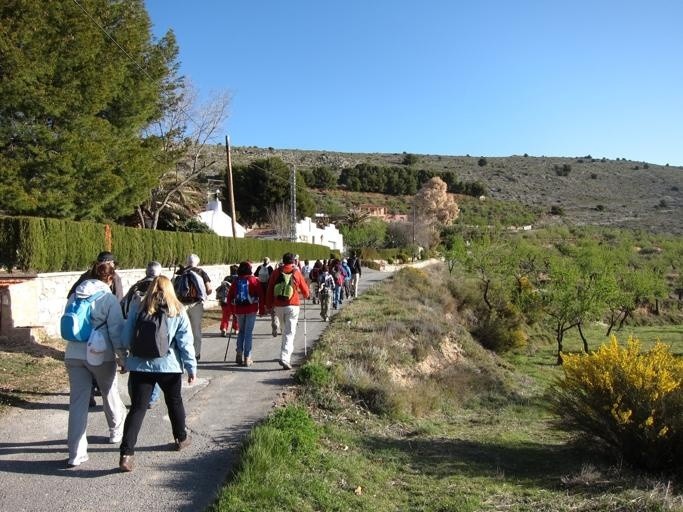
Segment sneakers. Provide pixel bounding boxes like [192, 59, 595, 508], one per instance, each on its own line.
[175, 435, 193, 452]
[235, 354, 242, 363]
[244, 356, 254, 367]
[69, 455, 89, 466]
[119, 454, 135, 473]
[221, 328, 225, 336]
[279, 359, 292, 368]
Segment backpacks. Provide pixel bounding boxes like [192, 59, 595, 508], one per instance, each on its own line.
[230, 277, 259, 306]
[258, 264, 270, 283]
[61, 291, 104, 342]
[215, 281, 230, 304]
[132, 301, 171, 358]
[273, 269, 301, 301]
[172, 266, 201, 303]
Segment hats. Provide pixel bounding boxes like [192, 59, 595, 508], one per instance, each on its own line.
[98, 251, 119, 262]
[86, 328, 106, 366]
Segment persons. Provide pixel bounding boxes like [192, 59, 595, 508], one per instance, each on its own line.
[61, 250, 363, 473]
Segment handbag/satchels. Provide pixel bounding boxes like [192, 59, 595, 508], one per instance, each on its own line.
[320, 287, 333, 297]
